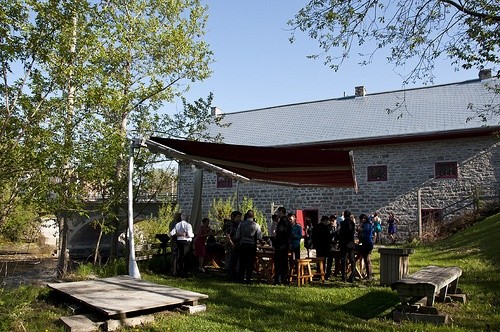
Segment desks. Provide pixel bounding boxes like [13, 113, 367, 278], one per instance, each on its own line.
[332, 250, 362, 276]
[204, 242, 225, 270]
[378, 248, 414, 286]
[257, 252, 292, 283]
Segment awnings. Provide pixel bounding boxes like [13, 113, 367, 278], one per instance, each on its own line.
[128, 136, 358, 279]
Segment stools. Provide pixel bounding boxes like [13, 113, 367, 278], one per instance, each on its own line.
[290, 258, 325, 285]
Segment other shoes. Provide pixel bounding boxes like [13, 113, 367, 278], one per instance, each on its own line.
[341, 277, 346, 282]
[350, 276, 356, 283]
[198, 266, 206, 272]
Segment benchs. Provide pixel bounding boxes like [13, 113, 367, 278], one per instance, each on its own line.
[390, 265, 466, 324]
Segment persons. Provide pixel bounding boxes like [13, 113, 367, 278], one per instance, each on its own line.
[169, 207, 398, 286]
